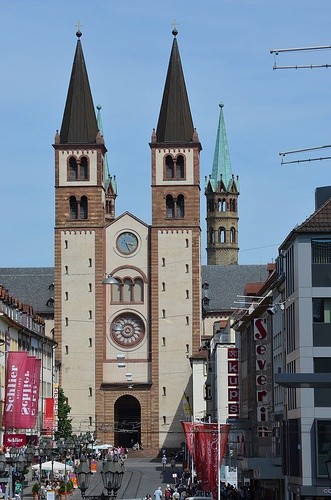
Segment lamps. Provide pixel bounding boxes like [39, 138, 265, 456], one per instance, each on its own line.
[102, 275, 118, 285]
[48, 283, 55, 291]
[202, 281, 210, 290]
[202, 295, 210, 306]
[45, 297, 54, 307]
[267, 306, 278, 315]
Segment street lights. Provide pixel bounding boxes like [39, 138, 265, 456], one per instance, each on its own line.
[10, 432, 100, 500]
[74, 454, 126, 500]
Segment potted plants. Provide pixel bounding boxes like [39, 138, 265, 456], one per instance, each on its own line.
[59, 480, 75, 500]
[31, 483, 40, 500]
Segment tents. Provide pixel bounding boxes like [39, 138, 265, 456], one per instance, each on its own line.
[32, 460, 73, 480]
[85, 443, 112, 453]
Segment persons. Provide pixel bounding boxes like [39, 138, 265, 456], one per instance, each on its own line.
[0, 446, 129, 500]
[133, 442, 260, 500]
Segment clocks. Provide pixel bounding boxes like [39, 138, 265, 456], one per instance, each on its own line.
[112, 228, 141, 257]
